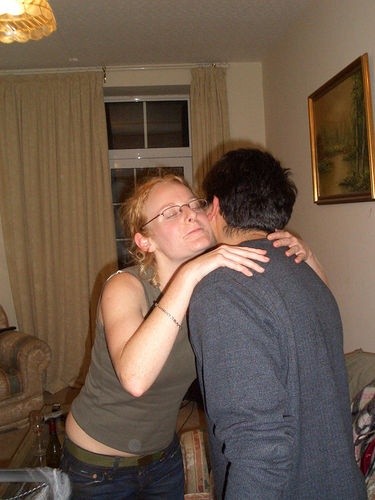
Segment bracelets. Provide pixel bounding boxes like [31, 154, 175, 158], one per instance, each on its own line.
[155, 303, 182, 329]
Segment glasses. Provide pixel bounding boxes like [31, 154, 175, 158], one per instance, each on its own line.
[143, 198, 208, 228]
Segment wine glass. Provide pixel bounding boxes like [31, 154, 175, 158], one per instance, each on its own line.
[30, 410, 46, 456]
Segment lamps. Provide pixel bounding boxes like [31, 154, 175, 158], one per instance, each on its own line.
[0, 0, 59, 46]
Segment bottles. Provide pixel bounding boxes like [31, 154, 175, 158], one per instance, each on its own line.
[48, 418, 61, 455]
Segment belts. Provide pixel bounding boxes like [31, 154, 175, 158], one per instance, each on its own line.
[64, 432, 164, 466]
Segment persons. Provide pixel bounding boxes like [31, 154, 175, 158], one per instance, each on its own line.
[60, 173, 328, 500]
[189, 147, 368, 500]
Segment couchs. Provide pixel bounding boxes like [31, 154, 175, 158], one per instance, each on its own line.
[178, 349, 375, 500]
[0, 305, 52, 433]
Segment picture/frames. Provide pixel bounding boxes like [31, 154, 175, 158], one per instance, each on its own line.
[308, 53, 375, 205]
